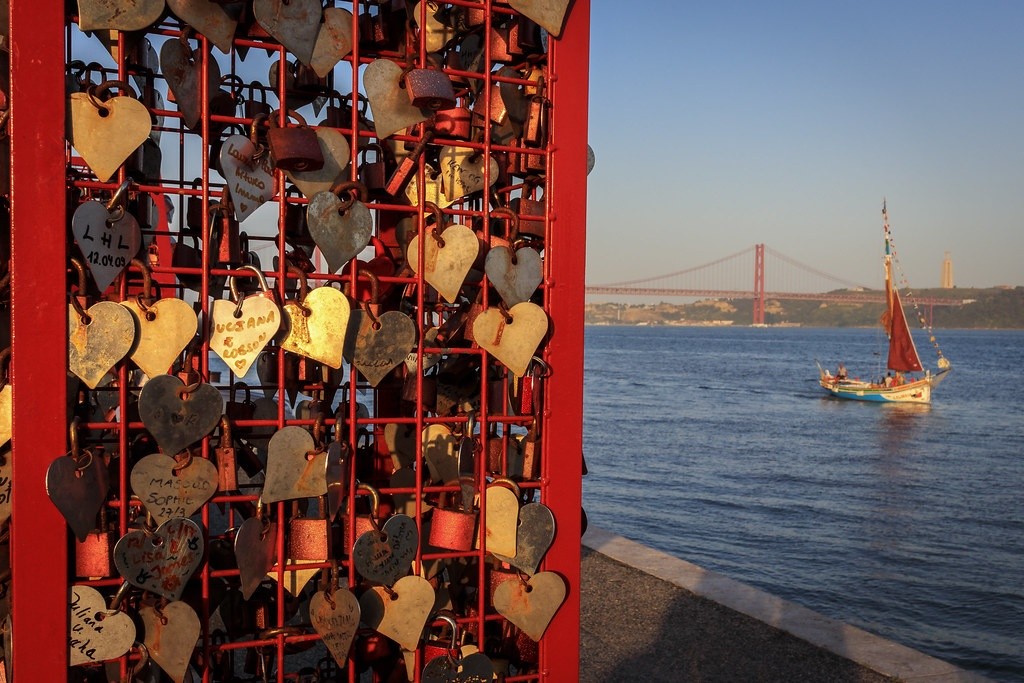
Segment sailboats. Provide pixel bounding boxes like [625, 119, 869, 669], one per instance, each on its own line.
[815, 193, 953, 403]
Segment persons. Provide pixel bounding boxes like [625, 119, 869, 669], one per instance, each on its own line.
[885, 371, 903, 388]
[835, 364, 848, 384]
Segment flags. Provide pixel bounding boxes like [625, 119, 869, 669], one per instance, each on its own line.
[888, 230, 943, 360]
